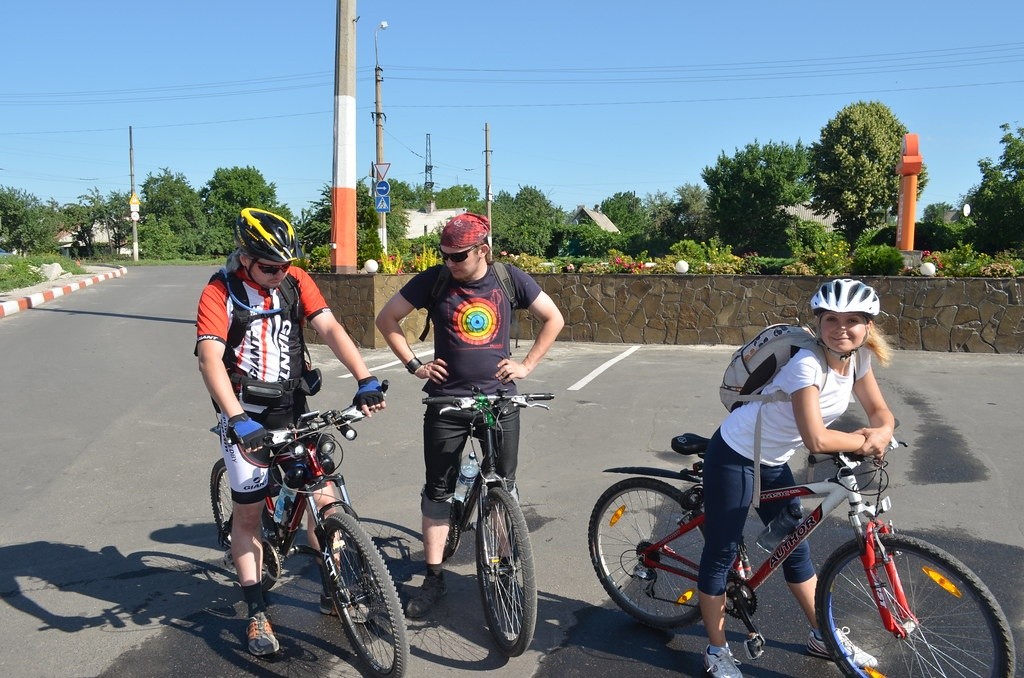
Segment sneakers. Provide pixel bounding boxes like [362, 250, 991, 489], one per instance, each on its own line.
[497, 570, 526, 609]
[806, 626, 878, 668]
[703, 642, 742, 678]
[320, 596, 369, 623]
[405, 573, 448, 618]
[245, 612, 280, 655]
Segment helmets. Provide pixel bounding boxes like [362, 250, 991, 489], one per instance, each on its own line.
[810, 279, 880, 316]
[235, 208, 303, 263]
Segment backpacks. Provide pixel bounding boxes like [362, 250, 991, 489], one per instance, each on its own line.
[718, 323, 857, 508]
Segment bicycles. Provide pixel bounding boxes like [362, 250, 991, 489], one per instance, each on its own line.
[419, 383, 555, 658]
[585, 432, 1017, 678]
[209, 377, 411, 678]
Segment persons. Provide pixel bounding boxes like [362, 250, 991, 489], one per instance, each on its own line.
[193, 207, 387, 657]
[375, 213, 564, 618]
[697, 277, 895, 678]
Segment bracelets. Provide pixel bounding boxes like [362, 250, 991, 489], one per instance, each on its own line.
[405, 357, 424, 374]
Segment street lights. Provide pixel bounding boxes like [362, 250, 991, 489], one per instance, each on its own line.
[371, 20, 390, 256]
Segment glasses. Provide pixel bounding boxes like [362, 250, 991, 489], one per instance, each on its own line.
[439, 247, 477, 262]
[257, 263, 291, 273]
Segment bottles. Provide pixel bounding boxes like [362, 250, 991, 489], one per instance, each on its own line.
[755, 503, 804, 553]
[272, 467, 302, 525]
[453, 452, 480, 502]
